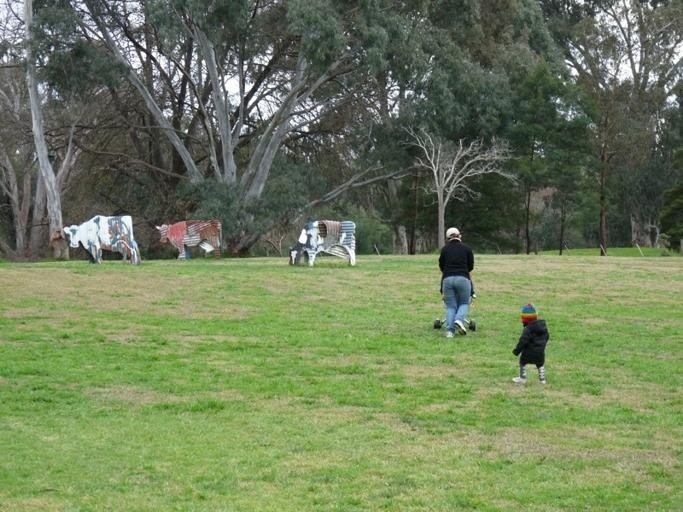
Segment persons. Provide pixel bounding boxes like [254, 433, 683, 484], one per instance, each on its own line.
[512, 303, 550, 387]
[439, 227, 475, 339]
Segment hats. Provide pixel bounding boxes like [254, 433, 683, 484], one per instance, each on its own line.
[446, 227, 460, 239]
[521, 302, 538, 322]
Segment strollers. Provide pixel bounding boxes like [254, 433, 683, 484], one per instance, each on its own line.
[430, 270, 480, 335]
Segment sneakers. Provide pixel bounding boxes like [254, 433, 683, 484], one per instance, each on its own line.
[512, 376, 527, 385]
[536, 378, 546, 385]
[445, 330, 455, 338]
[454, 320, 467, 335]
